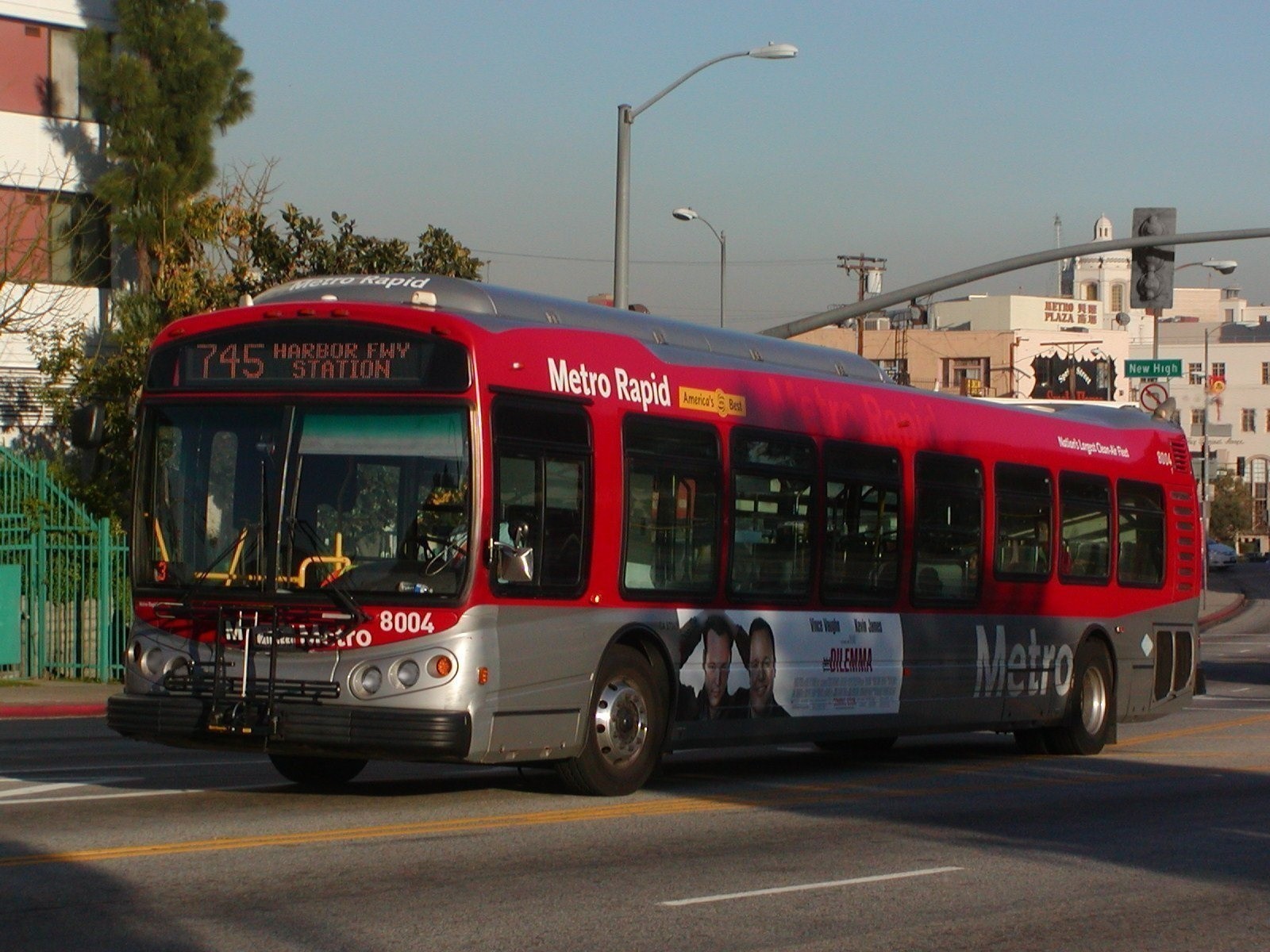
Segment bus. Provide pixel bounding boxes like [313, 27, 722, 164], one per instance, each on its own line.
[68, 277, 1207, 797]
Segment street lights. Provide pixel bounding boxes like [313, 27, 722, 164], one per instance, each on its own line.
[673, 209, 726, 331]
[611, 42, 800, 314]
[1149, 258, 1237, 377]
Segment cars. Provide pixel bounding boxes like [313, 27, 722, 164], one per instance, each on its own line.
[1207, 536, 1237, 570]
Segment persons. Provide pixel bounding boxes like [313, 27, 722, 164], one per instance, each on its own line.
[443, 488, 516, 583]
[677, 609, 753, 722]
[1033, 516, 1072, 575]
[965, 550, 979, 582]
[739, 616, 792, 719]
